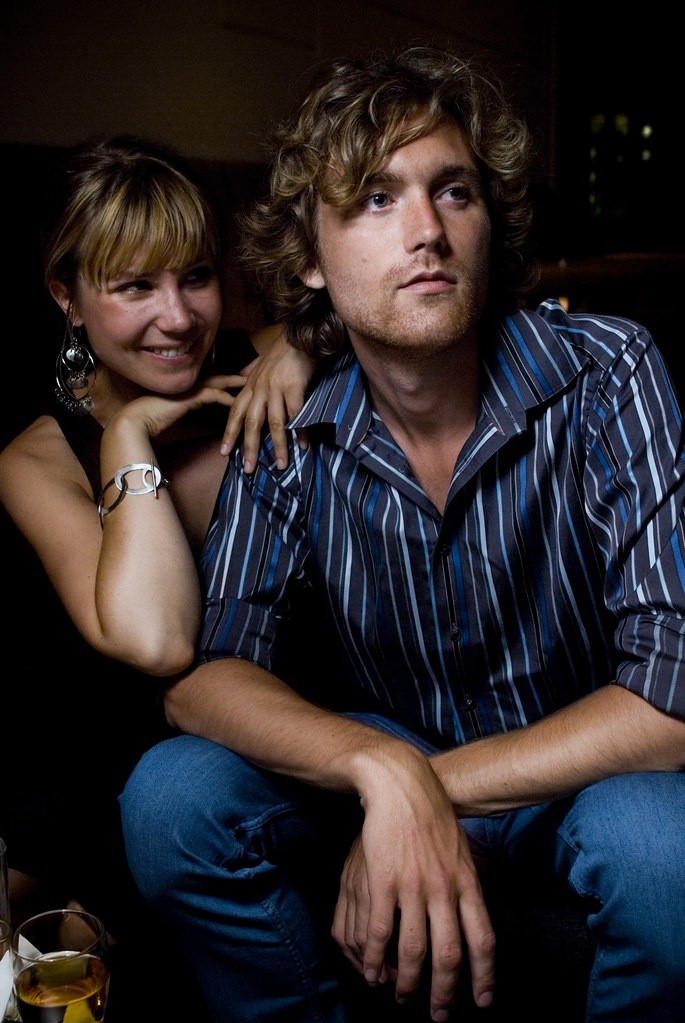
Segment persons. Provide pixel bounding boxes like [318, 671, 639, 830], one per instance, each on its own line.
[115, 41, 685, 1023]
[0, 135, 318, 1023]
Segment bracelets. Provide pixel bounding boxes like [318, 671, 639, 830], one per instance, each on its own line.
[97, 458, 171, 528]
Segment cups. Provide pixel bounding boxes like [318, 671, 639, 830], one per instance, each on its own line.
[9, 909, 111, 1023]
[0, 918, 22, 1023]
